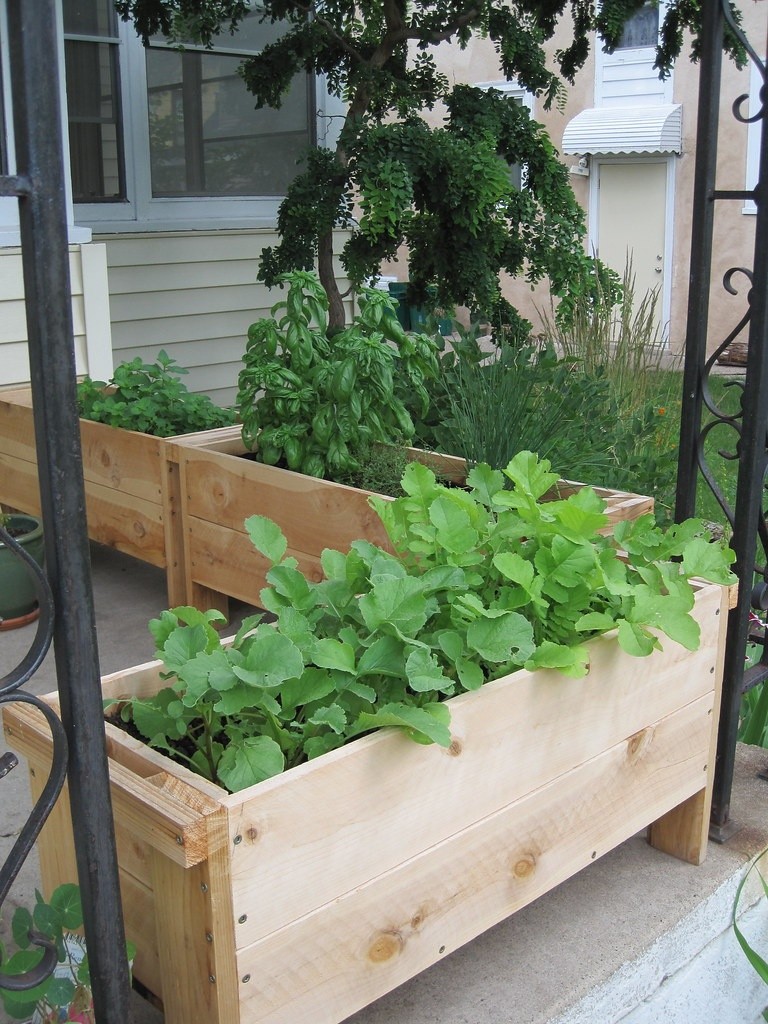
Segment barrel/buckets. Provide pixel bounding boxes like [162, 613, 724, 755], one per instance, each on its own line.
[380, 282, 411, 330]
[410, 286, 452, 336]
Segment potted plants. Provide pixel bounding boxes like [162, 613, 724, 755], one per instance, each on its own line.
[165, 268, 656, 628]
[2, 451, 739, 1024]
[1, 349, 244, 618]
